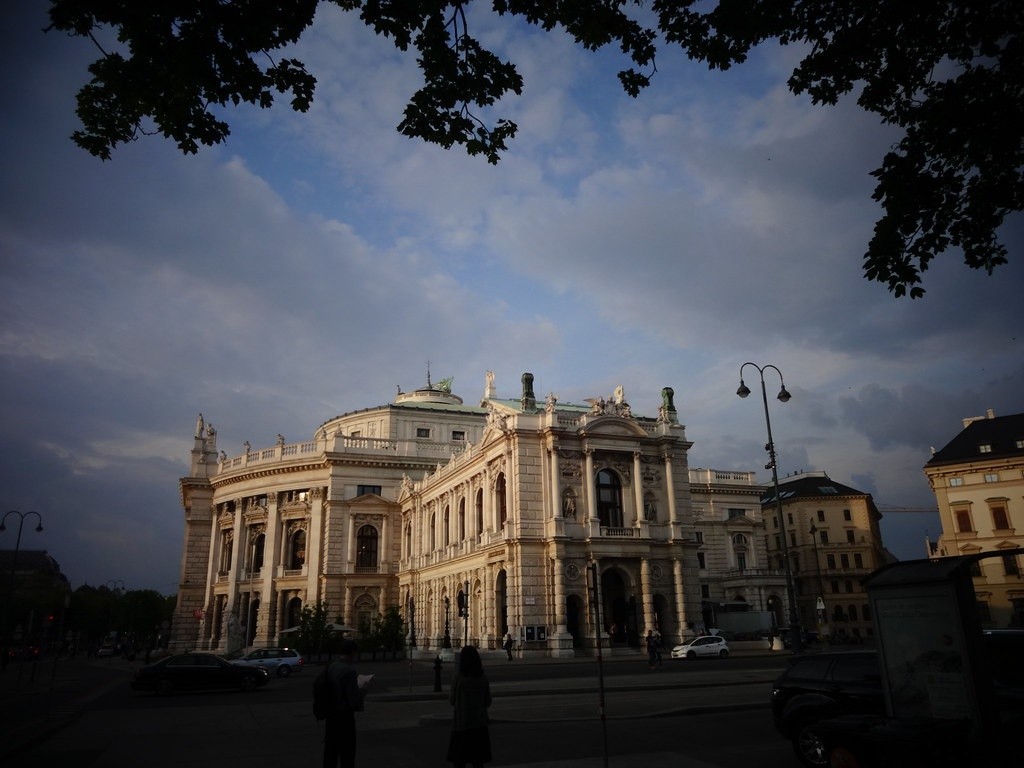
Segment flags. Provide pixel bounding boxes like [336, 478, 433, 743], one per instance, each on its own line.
[193, 610, 201, 619]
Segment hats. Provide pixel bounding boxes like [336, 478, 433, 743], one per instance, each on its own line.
[655, 634, 660, 637]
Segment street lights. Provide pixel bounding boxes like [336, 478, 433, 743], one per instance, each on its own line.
[441, 596, 453, 649]
[736, 361, 810, 657]
[102, 578, 128, 637]
[1, 510, 44, 622]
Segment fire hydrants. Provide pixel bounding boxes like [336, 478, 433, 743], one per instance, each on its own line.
[434, 655, 445, 693]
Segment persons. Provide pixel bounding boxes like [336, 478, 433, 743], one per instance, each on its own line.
[699, 628, 712, 636]
[505, 634, 513, 661]
[313, 645, 375, 768]
[607, 622, 664, 669]
[447, 645, 493, 768]
[195, 369, 659, 524]
[88, 639, 136, 664]
[768, 632, 774, 650]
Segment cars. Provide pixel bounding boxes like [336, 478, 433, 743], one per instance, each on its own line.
[671, 635, 731, 659]
[130, 652, 272, 698]
[226, 646, 304, 678]
[766, 627, 1024, 767]
[98, 644, 114, 657]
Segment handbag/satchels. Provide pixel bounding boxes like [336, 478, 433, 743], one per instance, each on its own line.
[312, 673, 332, 721]
